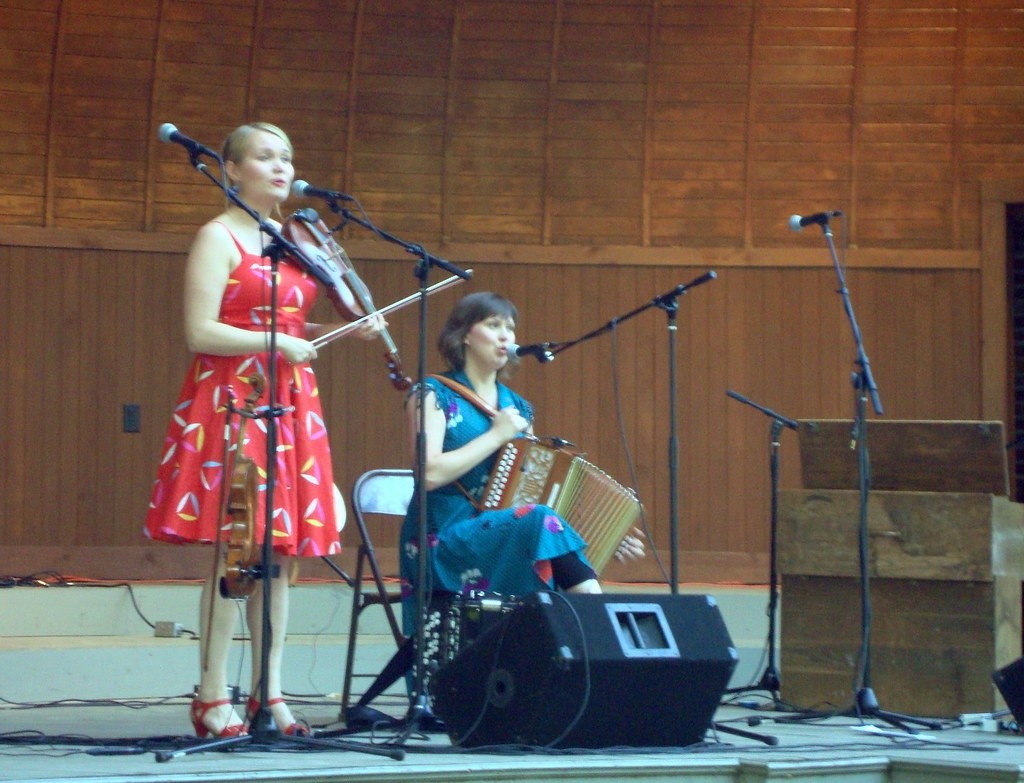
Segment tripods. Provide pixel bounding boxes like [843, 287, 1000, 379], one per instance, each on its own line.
[722, 223, 944, 735]
[153, 155, 473, 763]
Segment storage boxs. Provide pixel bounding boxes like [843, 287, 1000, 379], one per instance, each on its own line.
[774, 422, 1023, 723]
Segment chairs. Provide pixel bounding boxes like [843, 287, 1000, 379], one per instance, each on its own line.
[340, 467, 418, 733]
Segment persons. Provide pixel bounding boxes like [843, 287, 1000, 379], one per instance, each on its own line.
[141, 120, 391, 743]
[397, 291, 647, 719]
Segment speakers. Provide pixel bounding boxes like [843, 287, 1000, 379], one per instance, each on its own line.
[428, 591, 738, 755]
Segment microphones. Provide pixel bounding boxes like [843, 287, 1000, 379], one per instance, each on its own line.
[505, 341, 553, 362]
[788, 210, 841, 232]
[291, 179, 354, 201]
[159, 122, 221, 162]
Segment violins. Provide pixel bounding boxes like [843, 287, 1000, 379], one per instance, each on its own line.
[279, 207, 415, 391]
[215, 370, 264, 600]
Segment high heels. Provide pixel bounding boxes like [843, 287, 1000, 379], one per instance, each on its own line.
[190, 699, 248, 737]
[246, 697, 309, 737]
